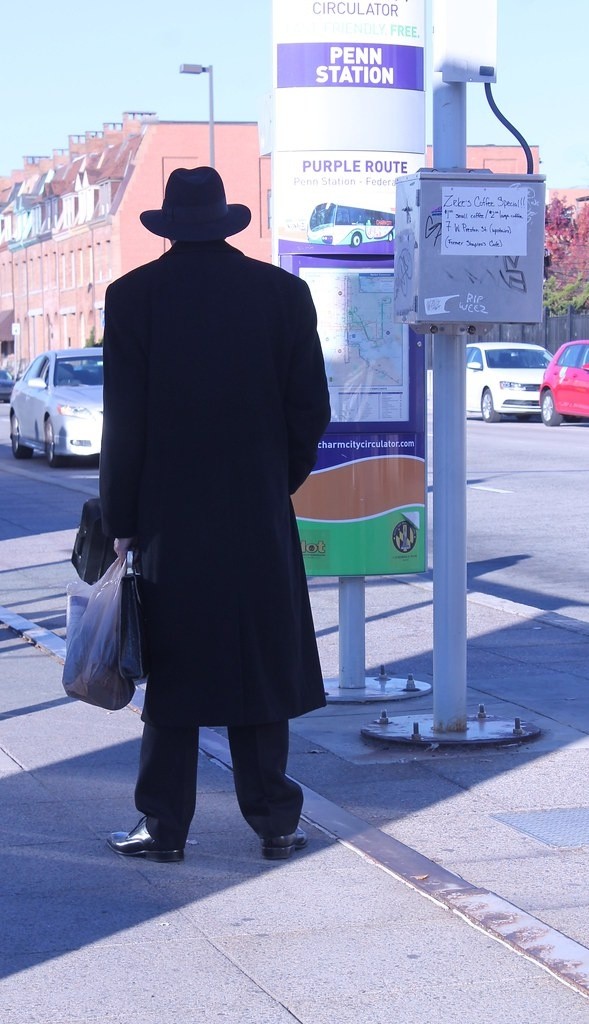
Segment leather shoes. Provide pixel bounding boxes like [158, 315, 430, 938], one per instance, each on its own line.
[261, 821, 309, 859]
[108, 817, 188, 862]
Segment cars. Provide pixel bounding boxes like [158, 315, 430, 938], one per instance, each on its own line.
[538, 339, 589, 427]
[0, 369, 15, 404]
[8, 347, 103, 468]
[465, 342, 555, 423]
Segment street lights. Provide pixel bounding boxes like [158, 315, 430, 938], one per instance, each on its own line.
[181, 63, 217, 169]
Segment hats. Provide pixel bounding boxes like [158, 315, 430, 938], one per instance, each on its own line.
[140, 167, 251, 239]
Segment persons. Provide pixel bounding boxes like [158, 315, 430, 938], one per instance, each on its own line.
[99, 166, 331, 863]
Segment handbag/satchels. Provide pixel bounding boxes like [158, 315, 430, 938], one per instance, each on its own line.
[72, 498, 133, 584]
[62, 550, 139, 709]
[116, 549, 147, 683]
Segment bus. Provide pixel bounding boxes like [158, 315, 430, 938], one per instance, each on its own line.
[306, 203, 396, 249]
